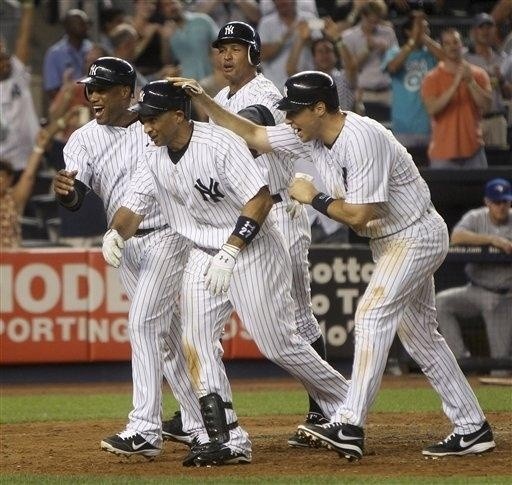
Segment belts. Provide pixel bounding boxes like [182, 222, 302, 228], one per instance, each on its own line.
[471, 280, 510, 295]
[482, 111, 502, 119]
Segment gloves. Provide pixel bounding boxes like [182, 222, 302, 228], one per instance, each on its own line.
[101, 228, 125, 269]
[285, 172, 313, 220]
[203, 243, 240, 296]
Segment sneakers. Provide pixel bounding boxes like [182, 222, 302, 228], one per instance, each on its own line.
[297, 421, 364, 462]
[287, 408, 324, 448]
[101, 429, 162, 458]
[161, 411, 193, 443]
[194, 442, 252, 466]
[421, 421, 496, 456]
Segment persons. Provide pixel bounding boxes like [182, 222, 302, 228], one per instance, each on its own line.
[100, 79, 351, 466]
[51, 56, 208, 458]
[285, 15, 359, 112]
[492, 1, 512, 21]
[0, 127, 51, 249]
[154, 19, 328, 448]
[167, 70, 498, 461]
[420, 25, 494, 169]
[124, 0, 163, 77]
[96, 7, 126, 57]
[57, 1, 113, 44]
[298, 0, 360, 31]
[160, 0, 220, 81]
[42, 8, 97, 101]
[462, 13, 512, 149]
[379, 10, 445, 146]
[340, 1, 399, 105]
[256, 0, 323, 98]
[44, 47, 110, 156]
[194, 0, 261, 32]
[108, 22, 182, 92]
[0, 0, 43, 169]
[436, 179, 512, 377]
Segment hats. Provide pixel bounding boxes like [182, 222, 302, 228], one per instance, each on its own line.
[485, 178, 512, 203]
[475, 13, 494, 28]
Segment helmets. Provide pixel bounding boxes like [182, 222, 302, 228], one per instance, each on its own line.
[76, 57, 135, 87]
[273, 71, 340, 112]
[212, 23, 261, 50]
[124, 80, 191, 117]
